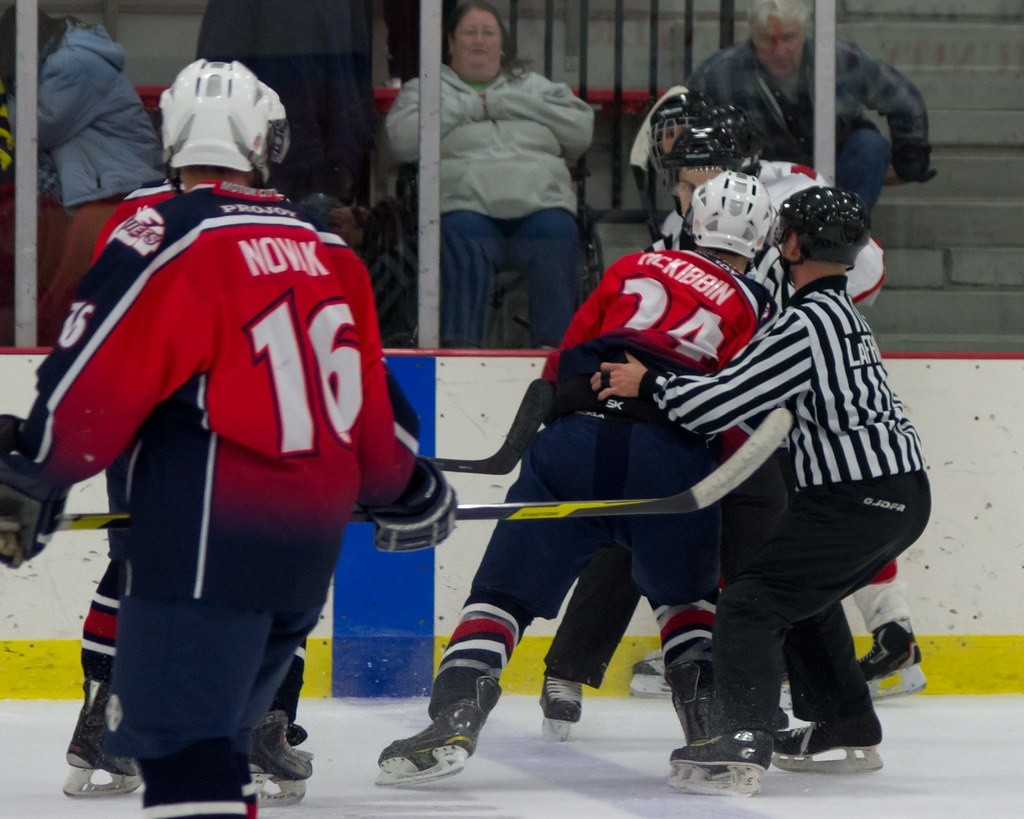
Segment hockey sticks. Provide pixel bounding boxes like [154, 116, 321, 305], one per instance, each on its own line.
[419, 377, 557, 476]
[2, 406, 792, 533]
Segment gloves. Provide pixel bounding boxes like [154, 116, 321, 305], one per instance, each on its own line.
[0, 452, 71, 571]
[371, 452, 461, 553]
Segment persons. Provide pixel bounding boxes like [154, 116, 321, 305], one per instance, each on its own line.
[687, 0, 940, 214]
[375, 98, 930, 794]
[0, 60, 457, 819]
[382, 0, 595, 355]
[1, 4, 166, 207]
[196, 0, 377, 211]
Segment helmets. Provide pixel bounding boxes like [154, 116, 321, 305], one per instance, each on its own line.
[650, 92, 762, 177]
[157, 58, 290, 192]
[772, 185, 872, 267]
[687, 170, 778, 260]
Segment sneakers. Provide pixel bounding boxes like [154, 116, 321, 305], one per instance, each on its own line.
[244, 710, 315, 808]
[64, 677, 141, 798]
[372, 674, 503, 790]
[767, 720, 885, 774]
[667, 716, 780, 797]
[538, 675, 584, 744]
[853, 619, 926, 700]
[630, 649, 676, 699]
[778, 675, 794, 712]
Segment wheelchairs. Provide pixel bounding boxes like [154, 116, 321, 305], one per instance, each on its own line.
[353, 102, 610, 342]
